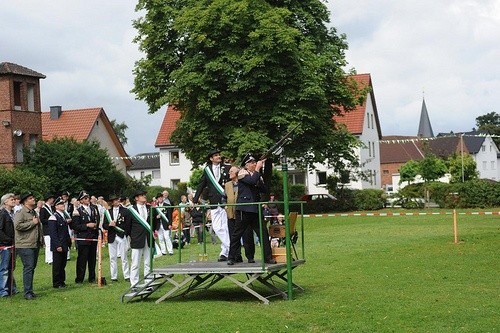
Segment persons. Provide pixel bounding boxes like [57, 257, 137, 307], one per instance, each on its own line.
[13, 192, 45, 300]
[273, 214, 294, 247]
[191, 149, 278, 264]
[0, 193, 20, 298]
[11, 195, 24, 214]
[34, 191, 108, 289]
[171, 193, 217, 249]
[102, 189, 174, 289]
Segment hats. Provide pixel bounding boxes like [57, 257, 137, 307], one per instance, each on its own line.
[54, 196, 66, 205]
[208, 149, 221, 158]
[18, 192, 33, 203]
[45, 194, 53, 201]
[155, 193, 163, 199]
[77, 191, 89, 201]
[133, 189, 148, 198]
[241, 153, 255, 166]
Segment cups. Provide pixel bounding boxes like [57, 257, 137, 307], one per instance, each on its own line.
[204, 255, 208, 261]
[199, 255, 202, 261]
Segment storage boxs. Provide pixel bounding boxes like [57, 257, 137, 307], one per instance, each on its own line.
[271, 246, 292, 263]
[269, 225, 286, 237]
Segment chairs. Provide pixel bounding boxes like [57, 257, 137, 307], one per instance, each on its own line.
[269, 212, 299, 260]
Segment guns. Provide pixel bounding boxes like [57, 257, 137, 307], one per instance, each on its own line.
[255, 122, 303, 163]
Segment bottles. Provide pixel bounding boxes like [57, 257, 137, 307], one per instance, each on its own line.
[104, 230, 108, 244]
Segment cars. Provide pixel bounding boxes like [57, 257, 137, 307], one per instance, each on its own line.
[384, 191, 426, 209]
[301, 193, 337, 201]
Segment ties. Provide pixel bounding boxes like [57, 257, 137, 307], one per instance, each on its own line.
[114, 209, 118, 222]
[141, 205, 146, 219]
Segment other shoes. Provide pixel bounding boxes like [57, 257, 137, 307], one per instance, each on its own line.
[218, 255, 227, 261]
[26, 291, 34, 300]
[264, 259, 277, 263]
[235, 257, 243, 262]
[146, 286, 153, 291]
[53, 283, 67, 288]
[227, 259, 234, 265]
[132, 287, 137, 292]
[248, 258, 255, 263]
